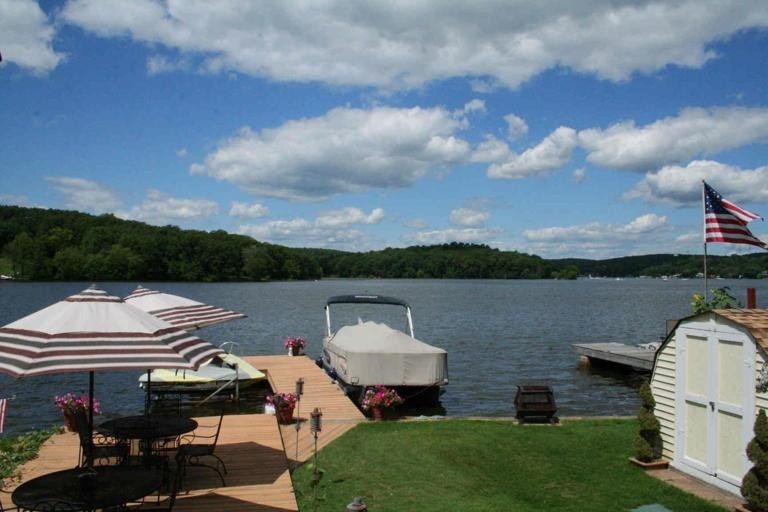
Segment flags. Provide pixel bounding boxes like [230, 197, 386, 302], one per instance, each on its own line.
[703, 180, 768, 250]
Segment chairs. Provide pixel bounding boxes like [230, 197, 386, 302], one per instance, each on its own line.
[0, 410, 228, 511]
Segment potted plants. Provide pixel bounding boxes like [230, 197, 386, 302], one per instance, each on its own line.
[734, 408, 768, 512]
[629, 381, 668, 470]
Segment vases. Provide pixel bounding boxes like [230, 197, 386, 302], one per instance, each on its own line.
[276, 408, 293, 424]
[290, 347, 298, 356]
[370, 406, 387, 420]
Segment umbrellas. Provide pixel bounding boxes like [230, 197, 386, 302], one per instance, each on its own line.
[120, 283, 248, 415]
[0, 280, 230, 468]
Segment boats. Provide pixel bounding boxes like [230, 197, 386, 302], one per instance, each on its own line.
[322, 294, 448, 392]
[137, 353, 267, 393]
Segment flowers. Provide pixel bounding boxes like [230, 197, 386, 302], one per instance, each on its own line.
[266, 392, 297, 417]
[361, 385, 405, 414]
[52, 389, 101, 417]
[283, 337, 305, 352]
